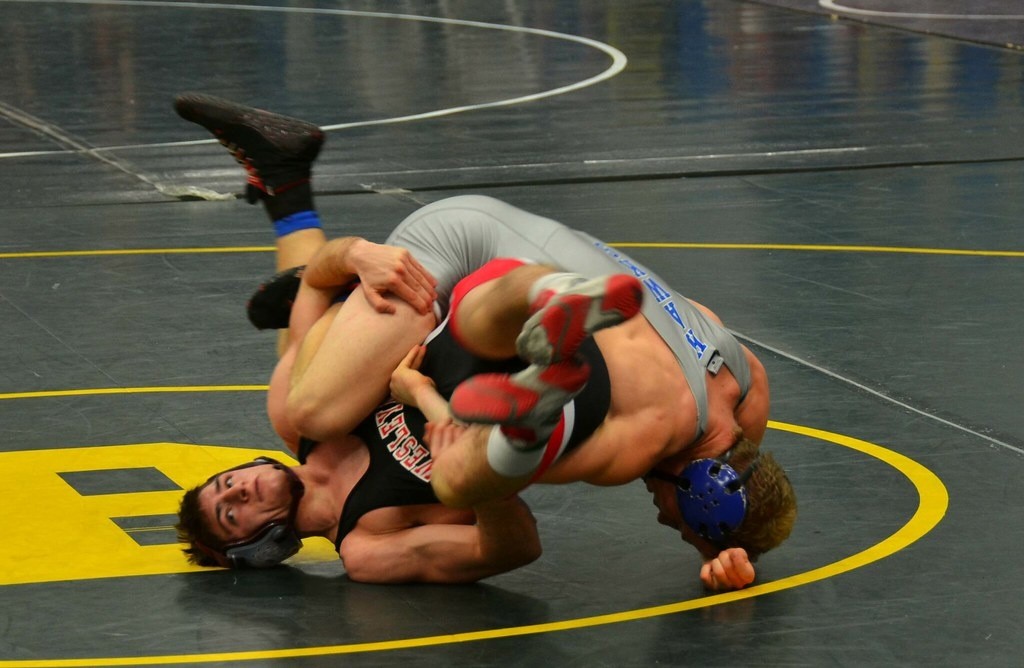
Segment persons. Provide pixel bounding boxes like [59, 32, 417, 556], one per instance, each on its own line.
[174, 236, 644, 584]
[171, 92, 797, 590]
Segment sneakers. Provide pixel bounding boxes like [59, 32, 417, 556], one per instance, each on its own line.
[449, 355, 592, 444]
[247, 264, 361, 330]
[515, 274, 644, 364]
[171, 90, 326, 205]
